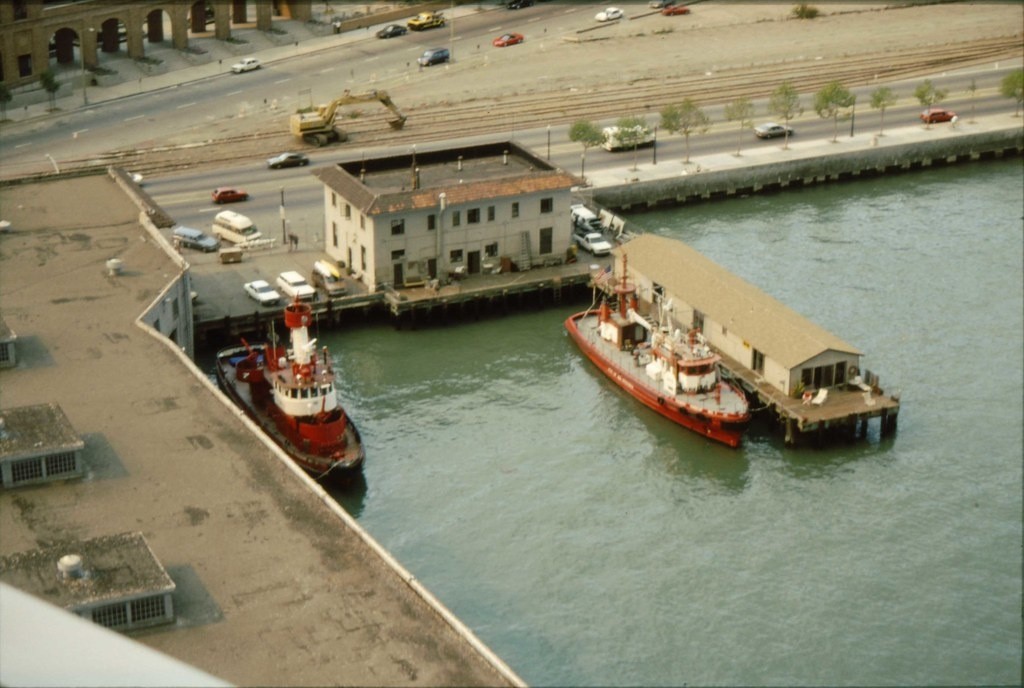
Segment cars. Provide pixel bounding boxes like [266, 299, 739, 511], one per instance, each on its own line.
[920, 108, 960, 123]
[753, 122, 794, 139]
[244, 280, 281, 306]
[232, 58, 262, 73]
[267, 153, 309, 168]
[376, 24, 408, 39]
[493, 32, 524, 47]
[648, 0, 690, 16]
[407, 12, 446, 31]
[595, 7, 624, 22]
[211, 187, 249, 204]
[277, 271, 316, 299]
[508, 0, 534, 10]
[173, 226, 221, 253]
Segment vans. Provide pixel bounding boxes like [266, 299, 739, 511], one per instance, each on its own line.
[212, 210, 263, 244]
[311, 260, 348, 296]
[417, 47, 450, 66]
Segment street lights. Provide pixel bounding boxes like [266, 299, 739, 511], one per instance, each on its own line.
[581, 154, 585, 179]
[546, 125, 552, 160]
[78, 27, 95, 105]
[280, 186, 286, 244]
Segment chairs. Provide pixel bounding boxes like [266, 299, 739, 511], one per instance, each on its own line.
[811, 388, 829, 406]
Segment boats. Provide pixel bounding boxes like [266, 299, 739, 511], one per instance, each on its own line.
[216, 295, 366, 475]
[564, 252, 752, 447]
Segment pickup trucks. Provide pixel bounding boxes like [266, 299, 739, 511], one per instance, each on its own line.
[573, 232, 612, 256]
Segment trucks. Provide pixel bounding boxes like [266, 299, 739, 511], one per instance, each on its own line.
[570, 204, 604, 233]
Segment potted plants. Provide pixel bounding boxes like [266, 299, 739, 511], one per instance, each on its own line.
[791, 379, 804, 399]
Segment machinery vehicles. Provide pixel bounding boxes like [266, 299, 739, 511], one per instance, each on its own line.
[290, 89, 408, 147]
[600, 125, 655, 150]
